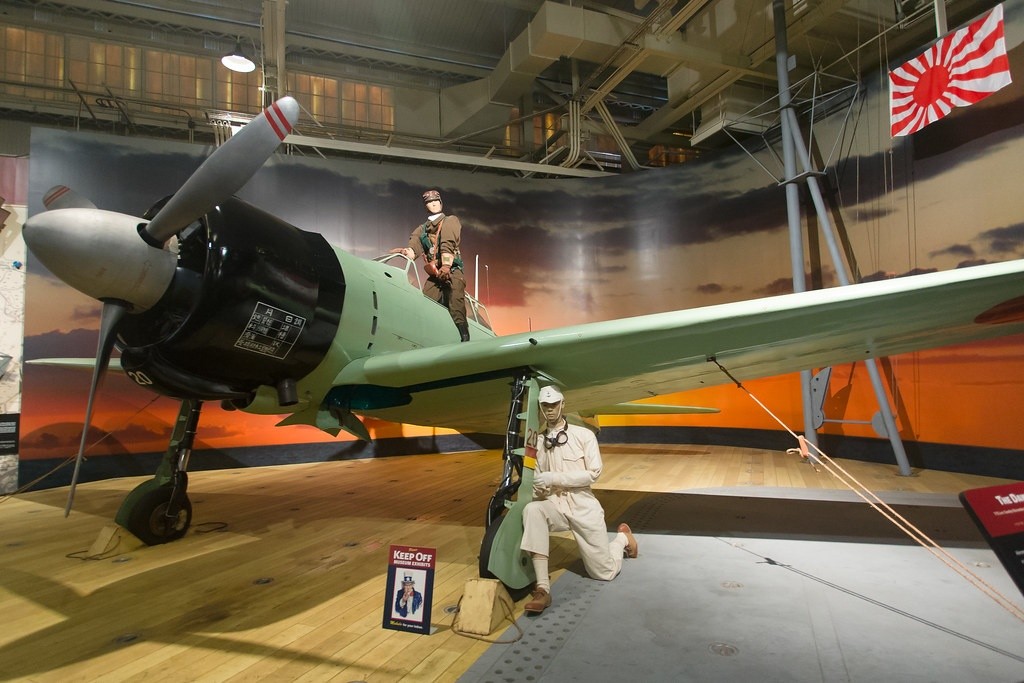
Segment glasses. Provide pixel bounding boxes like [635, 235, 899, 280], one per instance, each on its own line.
[538, 418, 569, 450]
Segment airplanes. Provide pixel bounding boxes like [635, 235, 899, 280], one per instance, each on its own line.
[19, 96, 1024, 571]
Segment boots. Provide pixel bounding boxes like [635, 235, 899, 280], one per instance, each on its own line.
[456, 323, 470, 342]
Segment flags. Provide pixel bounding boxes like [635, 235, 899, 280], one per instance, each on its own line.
[888, 3, 1012, 138]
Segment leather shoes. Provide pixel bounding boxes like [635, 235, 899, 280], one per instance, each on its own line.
[617, 523, 638, 558]
[525, 587, 552, 612]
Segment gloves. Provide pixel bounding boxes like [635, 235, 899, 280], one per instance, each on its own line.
[390, 248, 407, 256]
[437, 266, 451, 280]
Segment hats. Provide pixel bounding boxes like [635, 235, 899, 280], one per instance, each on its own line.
[401, 571, 415, 585]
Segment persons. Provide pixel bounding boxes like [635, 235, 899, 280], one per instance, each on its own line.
[520, 384, 638, 612]
[389, 190, 470, 342]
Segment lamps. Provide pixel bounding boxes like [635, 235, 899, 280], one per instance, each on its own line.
[222, 0, 255, 73]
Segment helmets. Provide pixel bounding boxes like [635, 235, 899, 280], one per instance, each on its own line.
[423, 191, 441, 203]
[538, 384, 565, 404]
